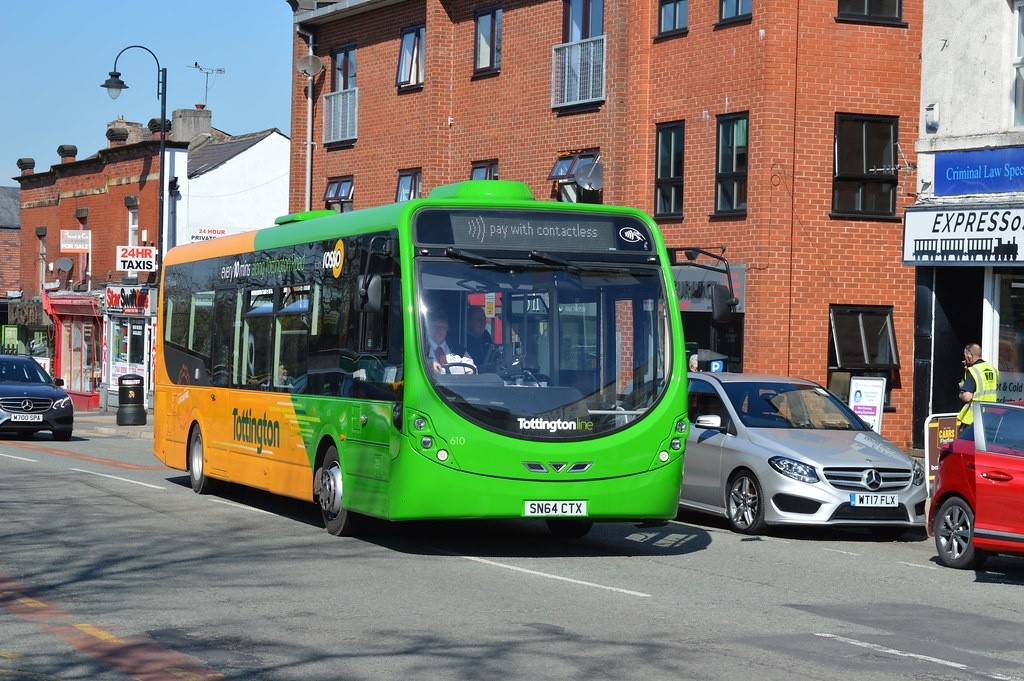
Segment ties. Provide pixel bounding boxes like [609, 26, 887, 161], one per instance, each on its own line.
[436, 346, 447, 365]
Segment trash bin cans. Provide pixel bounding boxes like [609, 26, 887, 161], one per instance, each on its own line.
[116, 374, 148, 426]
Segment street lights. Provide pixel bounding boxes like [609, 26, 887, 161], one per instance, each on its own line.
[100, 45, 167, 307]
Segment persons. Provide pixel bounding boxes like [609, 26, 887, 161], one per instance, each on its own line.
[688, 354, 699, 372]
[957, 344, 999, 439]
[465, 305, 500, 373]
[426, 311, 478, 375]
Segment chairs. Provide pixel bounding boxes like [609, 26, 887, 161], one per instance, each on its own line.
[698, 394, 727, 422]
[752, 393, 785, 427]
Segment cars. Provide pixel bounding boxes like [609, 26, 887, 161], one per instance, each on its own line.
[0, 354, 74, 441]
[615, 372, 928, 538]
[924, 400, 1024, 570]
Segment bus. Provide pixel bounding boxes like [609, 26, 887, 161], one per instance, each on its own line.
[153, 180, 739, 537]
